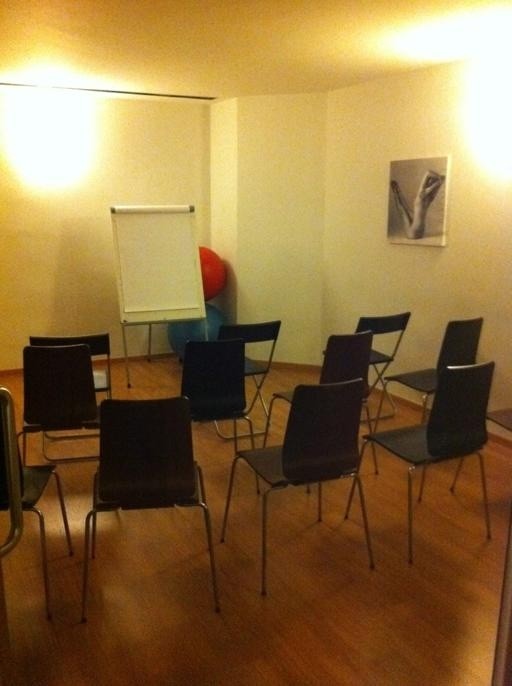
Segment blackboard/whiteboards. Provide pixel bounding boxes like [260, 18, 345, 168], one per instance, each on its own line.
[110, 205, 207, 327]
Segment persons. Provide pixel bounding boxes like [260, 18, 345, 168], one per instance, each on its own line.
[391, 171, 445, 239]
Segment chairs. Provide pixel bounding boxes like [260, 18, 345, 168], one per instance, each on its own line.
[81, 396, 221, 624]
[1, 387, 74, 624]
[344, 361, 496, 565]
[180, 311, 496, 498]
[21, 331, 111, 465]
[220, 377, 376, 596]
